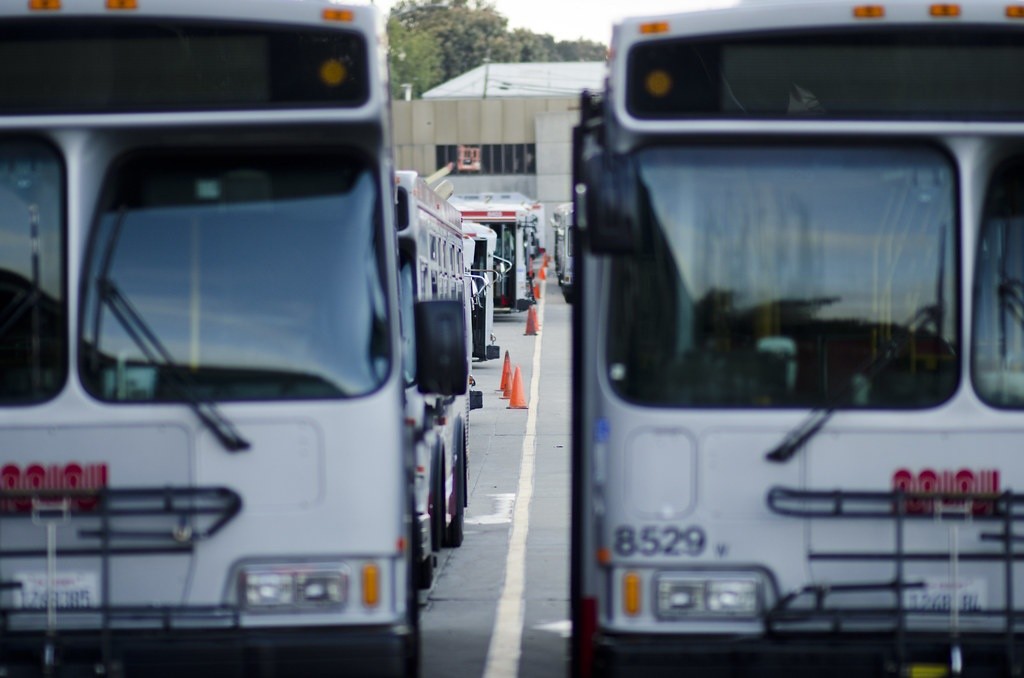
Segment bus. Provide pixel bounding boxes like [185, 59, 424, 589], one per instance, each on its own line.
[551, 202, 576, 306]
[568, 0, 1024, 678]
[395, 170, 484, 587]
[0, 0, 472, 678]
[461, 223, 512, 362]
[449, 193, 546, 317]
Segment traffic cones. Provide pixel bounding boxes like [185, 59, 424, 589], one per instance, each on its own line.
[523, 306, 538, 336]
[537, 267, 545, 279]
[499, 362, 513, 399]
[505, 365, 529, 409]
[542, 252, 548, 267]
[532, 305, 541, 331]
[533, 283, 540, 298]
[495, 350, 511, 390]
